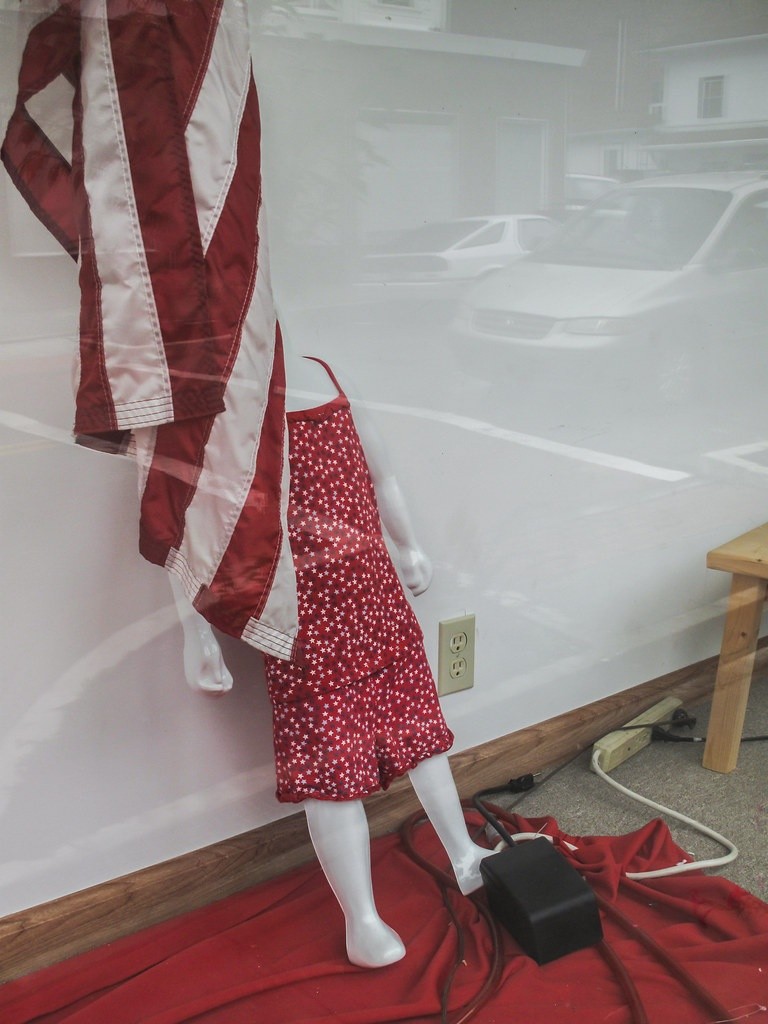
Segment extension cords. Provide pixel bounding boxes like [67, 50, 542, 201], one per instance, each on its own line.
[589, 697, 683, 775]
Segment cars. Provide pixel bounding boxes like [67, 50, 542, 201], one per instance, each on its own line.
[351, 209, 563, 310]
[452, 164, 768, 377]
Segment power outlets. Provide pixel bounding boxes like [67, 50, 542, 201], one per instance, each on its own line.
[439, 614, 475, 697]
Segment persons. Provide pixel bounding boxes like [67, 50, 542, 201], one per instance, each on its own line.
[169, 349, 503, 967]
